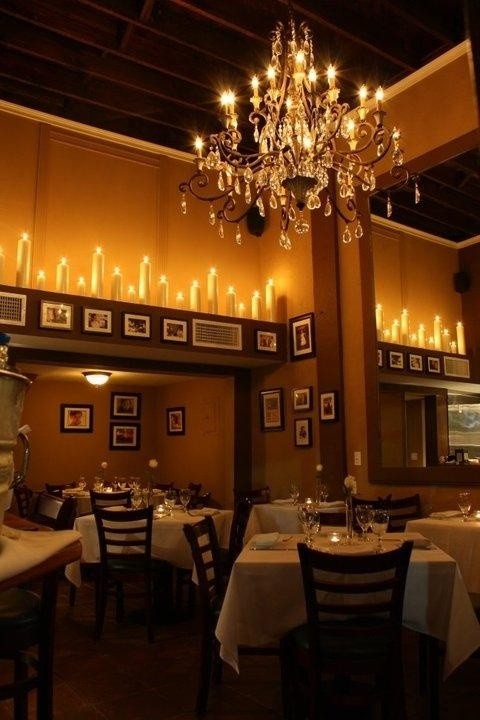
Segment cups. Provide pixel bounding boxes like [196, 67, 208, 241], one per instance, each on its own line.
[77, 474, 142, 492]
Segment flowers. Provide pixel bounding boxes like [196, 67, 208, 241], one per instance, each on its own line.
[342, 475, 357, 496]
[100, 460, 109, 472]
[312, 465, 325, 478]
[144, 459, 158, 478]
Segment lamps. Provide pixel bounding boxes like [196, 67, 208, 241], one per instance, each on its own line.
[169, 0, 426, 252]
[81, 370, 113, 387]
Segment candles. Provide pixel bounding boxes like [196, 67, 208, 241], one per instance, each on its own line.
[373, 302, 468, 357]
[0, 230, 277, 324]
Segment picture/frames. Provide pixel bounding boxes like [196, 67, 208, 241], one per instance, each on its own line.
[258, 385, 340, 448]
[287, 311, 316, 361]
[59, 390, 187, 452]
[36, 297, 191, 347]
[256, 328, 279, 352]
[376, 347, 443, 377]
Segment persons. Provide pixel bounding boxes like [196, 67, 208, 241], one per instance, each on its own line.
[323, 396, 334, 416]
[297, 393, 306, 404]
[67, 409, 87, 427]
[298, 425, 307, 443]
[295, 326, 309, 350]
[117, 398, 134, 414]
[170, 414, 181, 429]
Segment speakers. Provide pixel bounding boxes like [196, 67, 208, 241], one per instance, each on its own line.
[454, 272, 470, 292]
[247, 207, 265, 237]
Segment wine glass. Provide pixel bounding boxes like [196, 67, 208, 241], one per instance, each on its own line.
[296, 501, 386, 553]
[454, 490, 472, 521]
[290, 478, 330, 505]
[130, 488, 192, 517]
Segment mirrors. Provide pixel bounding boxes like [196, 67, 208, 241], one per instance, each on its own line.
[351, 124, 479, 487]
